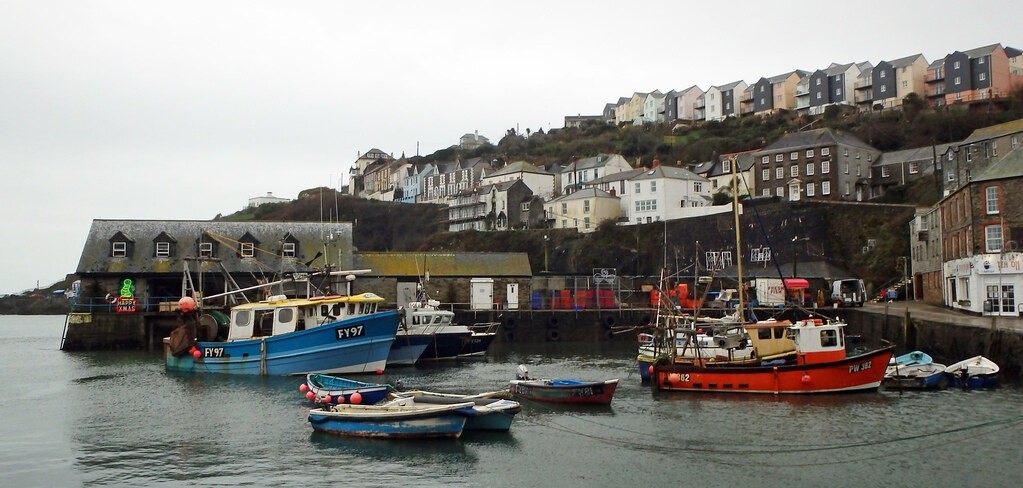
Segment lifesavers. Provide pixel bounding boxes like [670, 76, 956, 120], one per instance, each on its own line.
[502, 316, 518, 329]
[548, 315, 559, 327]
[547, 328, 560, 341]
[104, 293, 117, 304]
[504, 330, 517, 343]
[605, 330, 617, 342]
[641, 314, 652, 323]
[604, 315, 617, 328]
[802, 319, 823, 326]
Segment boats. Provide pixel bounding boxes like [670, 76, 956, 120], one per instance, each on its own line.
[386, 254, 502, 366]
[375, 390, 521, 432]
[945, 355, 999, 390]
[162, 185, 405, 377]
[308, 401, 476, 442]
[508, 364, 620, 406]
[885, 350, 932, 374]
[884, 362, 946, 389]
[298, 373, 391, 406]
[637, 156, 897, 396]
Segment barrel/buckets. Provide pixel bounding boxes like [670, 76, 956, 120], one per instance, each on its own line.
[533, 288, 614, 309]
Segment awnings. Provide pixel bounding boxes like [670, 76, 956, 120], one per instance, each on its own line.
[784, 278, 809, 288]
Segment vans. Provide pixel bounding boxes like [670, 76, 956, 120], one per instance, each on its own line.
[831, 279, 868, 308]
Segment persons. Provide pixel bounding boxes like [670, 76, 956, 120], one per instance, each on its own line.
[881, 290, 888, 302]
